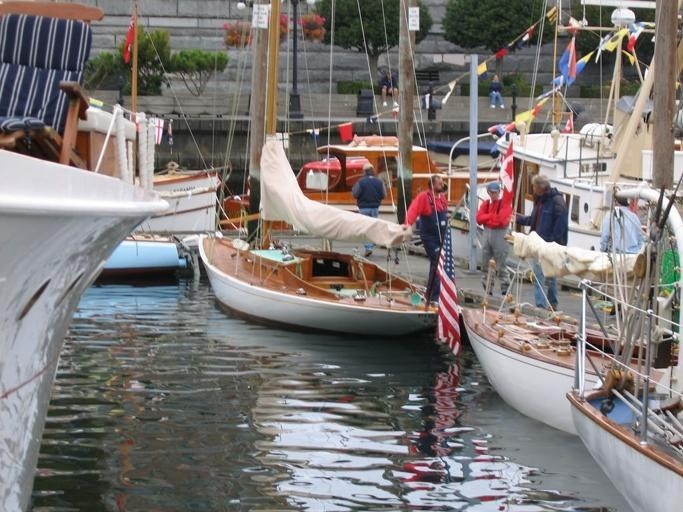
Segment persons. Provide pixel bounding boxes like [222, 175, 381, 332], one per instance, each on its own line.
[380, 68, 400, 107]
[403, 175, 448, 303]
[476, 182, 513, 298]
[351, 163, 387, 258]
[599, 196, 644, 316]
[509, 173, 569, 312]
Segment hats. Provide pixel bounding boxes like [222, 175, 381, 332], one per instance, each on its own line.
[486, 183, 500, 193]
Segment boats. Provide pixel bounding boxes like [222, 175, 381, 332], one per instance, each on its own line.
[0, 143, 175, 512]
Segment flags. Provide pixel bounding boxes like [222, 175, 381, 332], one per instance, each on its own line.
[155, 118, 164, 145]
[422, 6, 656, 138]
[339, 122, 353, 143]
[434, 224, 461, 356]
[498, 138, 514, 209]
[433, 362, 462, 456]
[123, 15, 135, 64]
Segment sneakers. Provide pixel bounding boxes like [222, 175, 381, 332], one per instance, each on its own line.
[364, 250, 372, 256]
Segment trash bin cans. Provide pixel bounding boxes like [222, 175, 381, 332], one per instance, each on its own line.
[356, 89, 374, 117]
